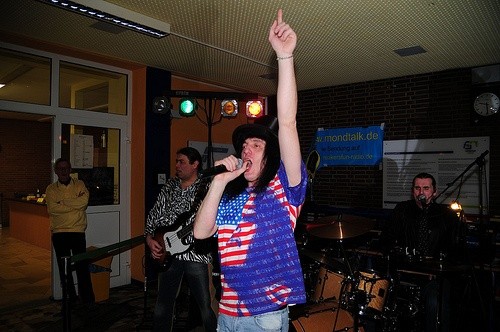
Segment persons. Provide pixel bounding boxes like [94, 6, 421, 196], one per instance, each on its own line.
[143, 146, 217, 332]
[376, 171, 468, 279]
[193, 9, 309, 332]
[45, 157, 96, 314]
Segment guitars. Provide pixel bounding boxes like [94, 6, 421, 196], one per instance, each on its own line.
[153, 213, 200, 259]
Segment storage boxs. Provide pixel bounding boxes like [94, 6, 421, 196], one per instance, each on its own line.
[87, 246, 113, 303]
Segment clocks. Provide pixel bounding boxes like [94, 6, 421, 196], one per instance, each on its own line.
[469, 84, 500, 127]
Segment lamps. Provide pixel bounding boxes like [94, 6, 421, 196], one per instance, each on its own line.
[153, 95, 170, 113]
[245, 100, 264, 117]
[221, 100, 238, 116]
[178, 97, 197, 115]
[41, 0, 170, 40]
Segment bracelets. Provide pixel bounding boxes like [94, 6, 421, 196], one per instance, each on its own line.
[277, 54, 293, 61]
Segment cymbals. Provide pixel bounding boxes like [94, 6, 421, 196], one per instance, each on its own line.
[297, 247, 348, 271]
[305, 214, 372, 239]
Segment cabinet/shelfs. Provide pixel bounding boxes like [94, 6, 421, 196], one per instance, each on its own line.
[5, 198, 50, 249]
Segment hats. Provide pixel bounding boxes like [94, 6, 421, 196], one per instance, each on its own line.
[232, 115, 279, 156]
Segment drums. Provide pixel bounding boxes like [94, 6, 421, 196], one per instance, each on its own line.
[351, 271, 391, 313]
[288, 300, 365, 332]
[311, 264, 353, 308]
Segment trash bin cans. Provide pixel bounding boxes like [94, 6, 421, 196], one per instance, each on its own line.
[91, 255, 113, 302]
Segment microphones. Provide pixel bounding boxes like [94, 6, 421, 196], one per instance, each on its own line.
[196, 158, 243, 179]
[418, 195, 427, 217]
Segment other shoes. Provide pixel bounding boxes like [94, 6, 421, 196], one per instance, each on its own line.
[190, 325, 205, 332]
